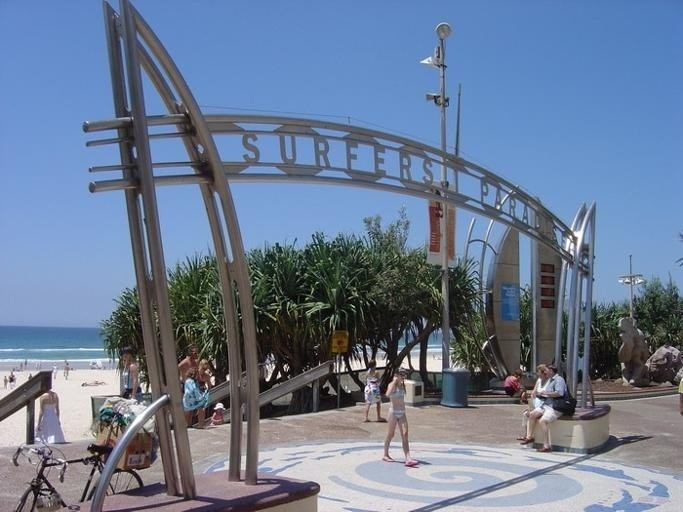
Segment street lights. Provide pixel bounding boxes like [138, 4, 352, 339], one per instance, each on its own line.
[421, 19, 457, 370]
[618, 253, 646, 317]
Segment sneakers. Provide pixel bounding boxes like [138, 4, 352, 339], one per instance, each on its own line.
[382, 454, 419, 466]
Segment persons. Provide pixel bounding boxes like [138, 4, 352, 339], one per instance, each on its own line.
[362, 359, 387, 423]
[209, 402, 226, 426]
[118, 345, 143, 403]
[194, 358, 212, 431]
[380, 367, 419, 467]
[177, 342, 199, 427]
[503, 368, 527, 407]
[35, 389, 66, 444]
[0, 356, 72, 394]
[515, 363, 558, 441]
[519, 364, 568, 453]
[676, 376, 682, 416]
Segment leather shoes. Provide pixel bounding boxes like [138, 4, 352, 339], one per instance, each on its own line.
[516, 436, 535, 445]
[538, 446, 552, 452]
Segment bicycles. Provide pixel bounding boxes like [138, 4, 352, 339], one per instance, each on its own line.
[11, 442, 145, 512]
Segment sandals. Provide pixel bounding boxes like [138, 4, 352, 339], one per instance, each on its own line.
[363, 417, 387, 422]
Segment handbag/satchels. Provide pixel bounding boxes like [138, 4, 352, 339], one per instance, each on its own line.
[552, 376, 578, 417]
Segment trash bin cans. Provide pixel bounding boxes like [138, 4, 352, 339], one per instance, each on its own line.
[440, 368, 470, 408]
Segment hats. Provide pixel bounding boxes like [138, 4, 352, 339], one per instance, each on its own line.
[213, 402, 226, 411]
